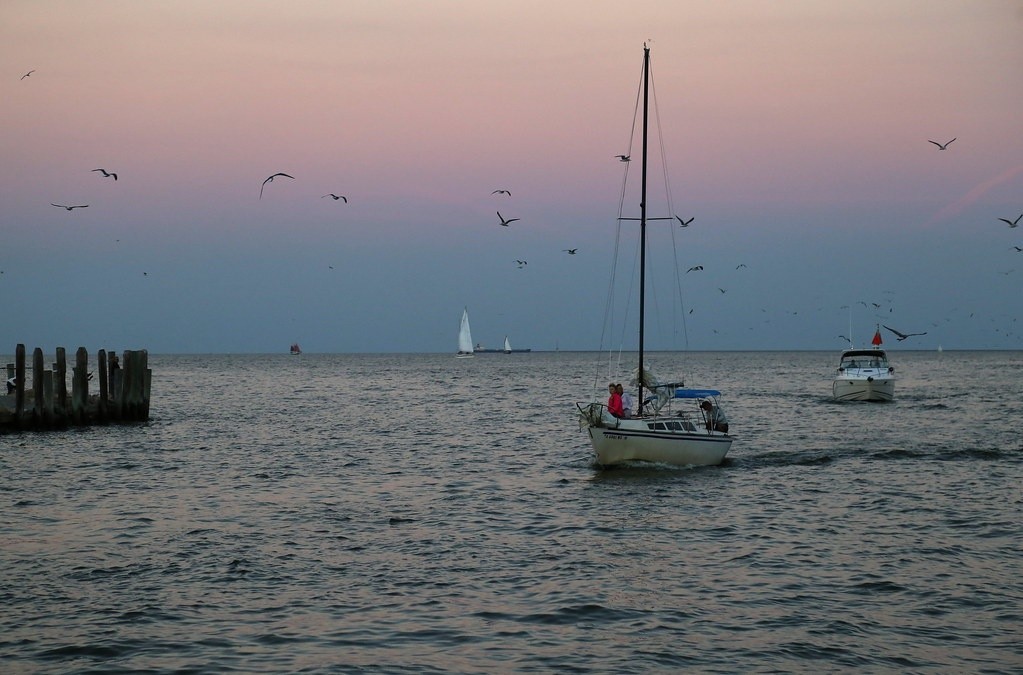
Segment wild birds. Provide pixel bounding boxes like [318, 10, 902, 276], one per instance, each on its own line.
[20, 70, 36, 80]
[674, 215, 697, 227]
[490, 190, 513, 197]
[998, 214, 1023, 229]
[91, 169, 118, 181]
[321, 193, 348, 203]
[1008, 247, 1023, 253]
[685, 261, 1023, 351]
[497, 211, 521, 227]
[563, 249, 578, 254]
[260, 173, 295, 200]
[927, 137, 957, 150]
[143, 272, 148, 276]
[513, 259, 528, 265]
[50, 202, 88, 211]
[614, 154, 631, 162]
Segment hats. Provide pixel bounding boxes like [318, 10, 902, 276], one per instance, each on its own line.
[700, 401, 710, 408]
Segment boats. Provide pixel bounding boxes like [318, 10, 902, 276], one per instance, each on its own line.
[473, 343, 531, 353]
[832, 347, 898, 405]
[290, 344, 301, 355]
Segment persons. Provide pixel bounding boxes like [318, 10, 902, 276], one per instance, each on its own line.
[607, 383, 623, 418]
[848, 360, 857, 367]
[109, 356, 119, 397]
[615, 383, 632, 419]
[699, 401, 728, 432]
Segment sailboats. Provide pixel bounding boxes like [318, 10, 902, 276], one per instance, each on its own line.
[455, 308, 475, 358]
[576, 40, 740, 471]
[503, 336, 512, 354]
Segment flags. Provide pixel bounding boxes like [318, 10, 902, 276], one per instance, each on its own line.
[871, 332, 881, 345]
